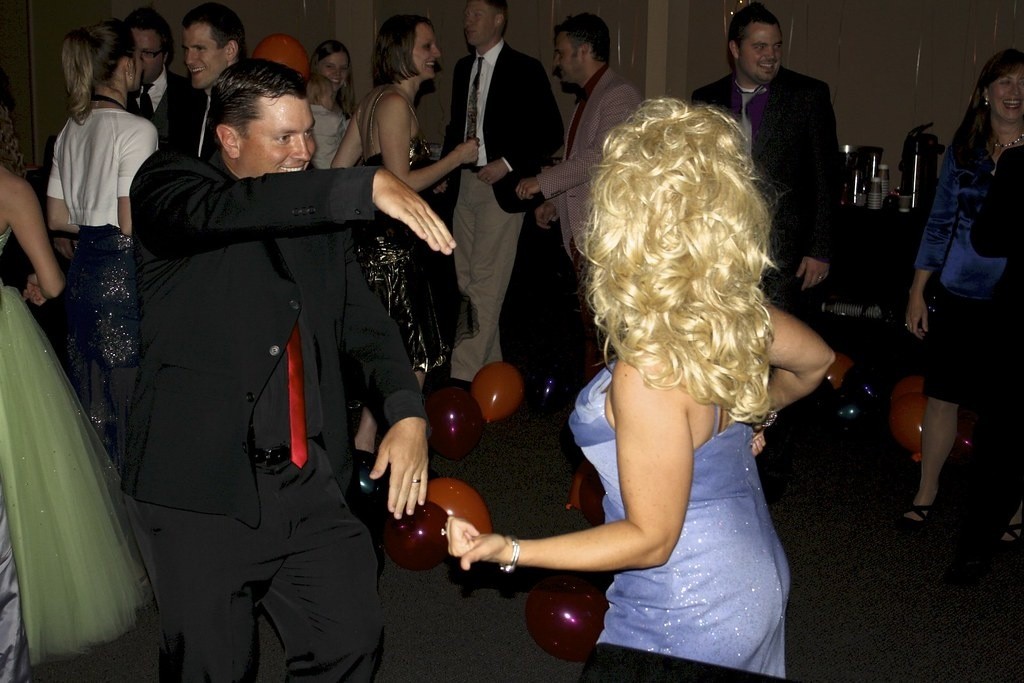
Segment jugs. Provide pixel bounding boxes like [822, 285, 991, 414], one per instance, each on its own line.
[898, 121, 945, 209]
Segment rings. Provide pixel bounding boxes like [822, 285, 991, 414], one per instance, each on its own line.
[522, 192, 525, 193]
[441, 528, 446, 536]
[819, 277, 822, 278]
[485, 178, 489, 181]
[905, 324, 907, 326]
[412, 479, 420, 483]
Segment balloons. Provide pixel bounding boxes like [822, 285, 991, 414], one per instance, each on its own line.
[252, 33, 310, 82]
[825, 353, 972, 462]
[346, 361, 612, 662]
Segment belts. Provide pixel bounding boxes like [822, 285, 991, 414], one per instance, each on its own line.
[247, 443, 291, 465]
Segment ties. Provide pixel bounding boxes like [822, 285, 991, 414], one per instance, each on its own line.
[286, 320, 309, 469]
[139, 83, 154, 119]
[468, 56, 480, 168]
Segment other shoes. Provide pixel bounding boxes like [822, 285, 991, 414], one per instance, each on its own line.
[896, 489, 939, 534]
[997, 518, 1021, 549]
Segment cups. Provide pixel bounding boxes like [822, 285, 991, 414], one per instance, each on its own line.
[867, 164, 890, 210]
[898, 195, 912, 212]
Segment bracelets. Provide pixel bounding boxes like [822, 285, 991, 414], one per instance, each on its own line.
[500, 536, 520, 573]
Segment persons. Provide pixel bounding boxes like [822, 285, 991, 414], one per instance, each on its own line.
[128, 56, 459, 683]
[0, 103, 158, 683]
[446, 96, 836, 683]
[900, 48, 1024, 586]
[47, 0, 841, 452]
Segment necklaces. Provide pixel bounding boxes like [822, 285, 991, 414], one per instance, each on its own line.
[994, 133, 1024, 147]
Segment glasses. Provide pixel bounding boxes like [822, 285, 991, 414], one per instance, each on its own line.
[139, 46, 163, 59]
